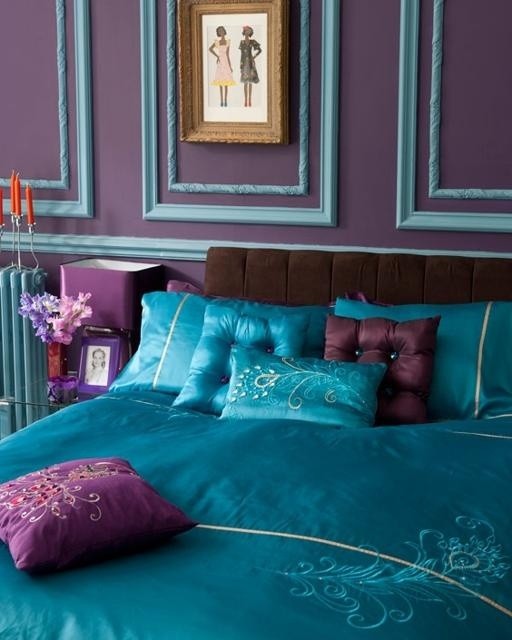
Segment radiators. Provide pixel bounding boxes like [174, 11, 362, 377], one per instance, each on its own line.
[0, 265, 49, 439]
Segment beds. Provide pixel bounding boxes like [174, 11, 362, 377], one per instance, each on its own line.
[0, 246, 511, 639]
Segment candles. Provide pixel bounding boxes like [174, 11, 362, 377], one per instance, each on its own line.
[0, 169, 34, 225]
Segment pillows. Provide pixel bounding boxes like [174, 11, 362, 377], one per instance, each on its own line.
[323, 313, 444, 423]
[168, 305, 311, 415]
[0, 456, 203, 578]
[219, 343, 388, 430]
[109, 279, 336, 395]
[335, 296, 510, 421]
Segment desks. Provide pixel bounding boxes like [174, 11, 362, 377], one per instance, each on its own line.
[0, 377, 80, 414]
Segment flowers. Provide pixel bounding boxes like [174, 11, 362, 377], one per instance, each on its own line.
[17, 291, 94, 346]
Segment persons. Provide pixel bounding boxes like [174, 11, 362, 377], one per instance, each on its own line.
[236, 25, 263, 107]
[208, 25, 235, 108]
[85, 348, 109, 385]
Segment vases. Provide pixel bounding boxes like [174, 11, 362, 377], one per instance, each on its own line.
[48, 342, 68, 378]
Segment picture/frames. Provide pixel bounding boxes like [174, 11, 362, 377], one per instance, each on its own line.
[76, 335, 123, 395]
[177, 0, 289, 144]
